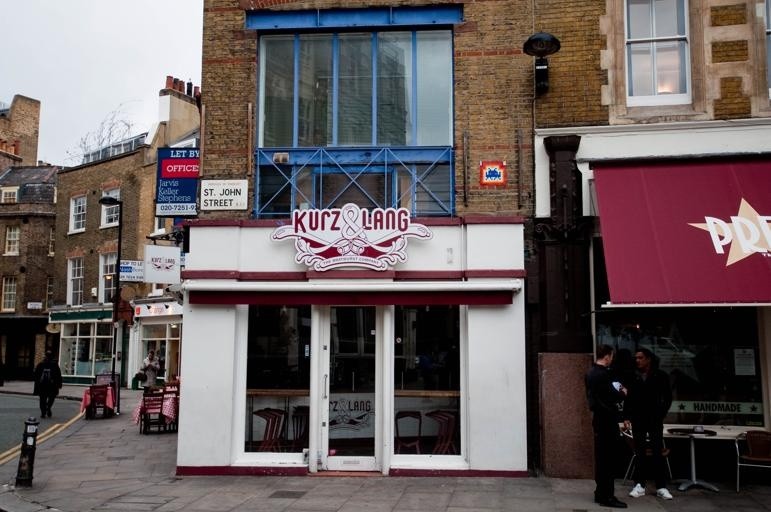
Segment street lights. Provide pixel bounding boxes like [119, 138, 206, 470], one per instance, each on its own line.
[99, 197, 123, 386]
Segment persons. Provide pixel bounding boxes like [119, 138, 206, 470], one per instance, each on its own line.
[33, 350, 63, 419]
[138, 350, 160, 393]
[618, 346, 677, 502]
[583, 342, 629, 510]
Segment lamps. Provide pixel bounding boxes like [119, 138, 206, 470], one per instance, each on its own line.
[523, 32, 561, 95]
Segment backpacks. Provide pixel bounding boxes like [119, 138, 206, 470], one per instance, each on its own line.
[40, 369, 55, 384]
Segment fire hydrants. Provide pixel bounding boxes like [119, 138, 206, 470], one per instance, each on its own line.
[15, 417, 39, 488]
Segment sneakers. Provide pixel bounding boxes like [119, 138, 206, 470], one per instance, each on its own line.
[656, 488, 672, 499]
[629, 483, 645, 498]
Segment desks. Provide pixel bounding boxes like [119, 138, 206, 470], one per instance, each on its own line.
[618, 423, 766, 492]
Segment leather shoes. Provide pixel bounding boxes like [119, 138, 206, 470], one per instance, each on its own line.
[595, 492, 627, 508]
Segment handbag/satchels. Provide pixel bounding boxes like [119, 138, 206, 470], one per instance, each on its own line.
[136, 373, 147, 381]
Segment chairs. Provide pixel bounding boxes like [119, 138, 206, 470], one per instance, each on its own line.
[88, 383, 180, 434]
[735, 430, 771, 493]
[620, 427, 672, 485]
